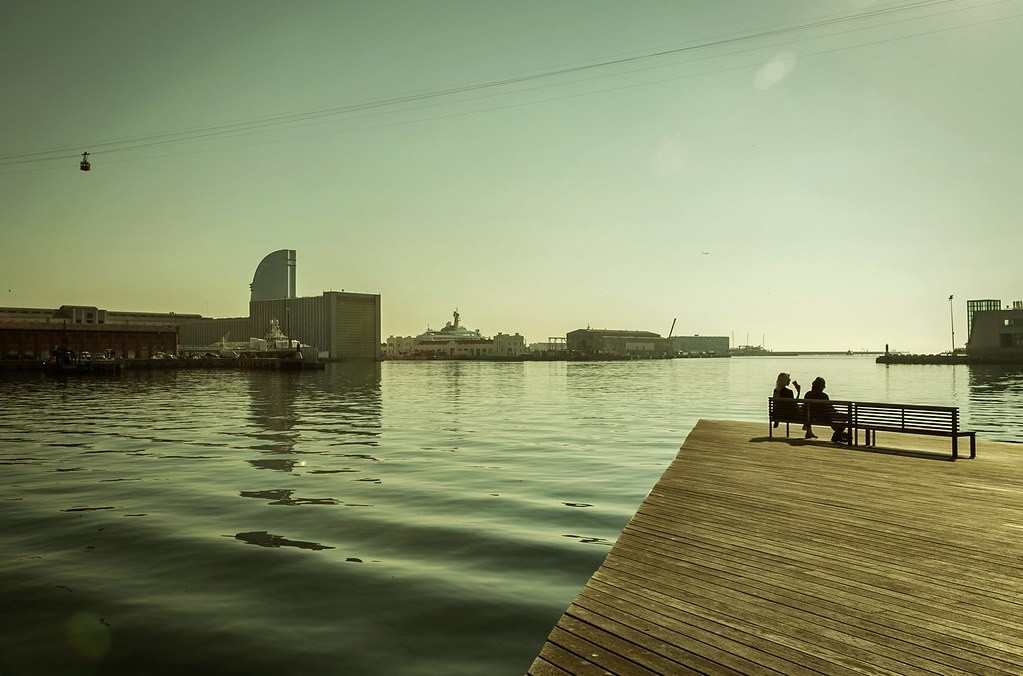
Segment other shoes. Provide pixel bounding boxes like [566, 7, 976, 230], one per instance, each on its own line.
[805, 432, 818, 439]
[831, 435, 848, 443]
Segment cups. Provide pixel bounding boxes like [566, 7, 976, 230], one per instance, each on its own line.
[792, 380, 798, 386]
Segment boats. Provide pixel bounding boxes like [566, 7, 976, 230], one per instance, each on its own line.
[221, 317, 318, 366]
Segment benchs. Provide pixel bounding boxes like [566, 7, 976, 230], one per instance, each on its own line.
[768, 397, 976, 459]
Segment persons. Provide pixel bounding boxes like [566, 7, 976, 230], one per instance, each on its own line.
[772, 373, 818, 439]
[804, 377, 853, 442]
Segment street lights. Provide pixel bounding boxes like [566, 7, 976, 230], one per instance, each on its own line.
[948, 295, 954, 349]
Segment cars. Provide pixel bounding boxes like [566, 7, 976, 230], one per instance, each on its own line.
[94, 353, 105, 359]
[79, 352, 90, 359]
[154, 352, 217, 359]
